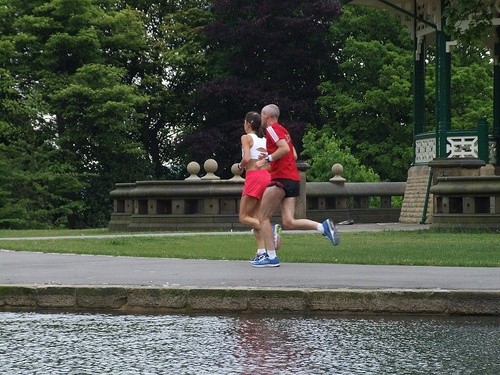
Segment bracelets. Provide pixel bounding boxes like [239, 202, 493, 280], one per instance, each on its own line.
[268, 155, 273, 161]
[238, 164, 242, 170]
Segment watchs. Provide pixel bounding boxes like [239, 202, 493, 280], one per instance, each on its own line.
[265, 157, 270, 163]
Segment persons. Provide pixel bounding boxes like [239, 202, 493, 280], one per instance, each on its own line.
[252, 104, 340, 268]
[231, 112, 282, 264]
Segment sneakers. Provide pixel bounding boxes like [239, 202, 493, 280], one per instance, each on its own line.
[252, 254, 280, 266]
[251, 252, 269, 263]
[272, 223, 282, 249]
[322, 218, 339, 246]
